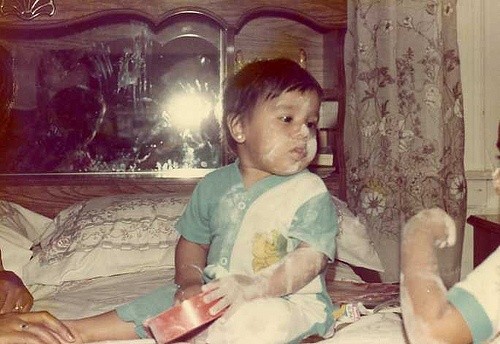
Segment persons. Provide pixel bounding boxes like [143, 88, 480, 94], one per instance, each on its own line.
[399, 122, 500, 344]
[0, 252, 76, 344]
[49, 58, 337, 344]
[0, 46, 107, 171]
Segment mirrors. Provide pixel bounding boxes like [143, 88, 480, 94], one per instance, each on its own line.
[0, 7, 223, 177]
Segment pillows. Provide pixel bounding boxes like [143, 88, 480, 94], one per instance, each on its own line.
[0, 197, 54, 270]
[23, 192, 384, 286]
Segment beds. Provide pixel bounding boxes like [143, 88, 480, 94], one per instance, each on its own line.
[0, 0, 407, 344]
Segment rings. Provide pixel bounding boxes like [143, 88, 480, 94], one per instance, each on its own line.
[20, 323, 29, 329]
[14, 304, 24, 310]
